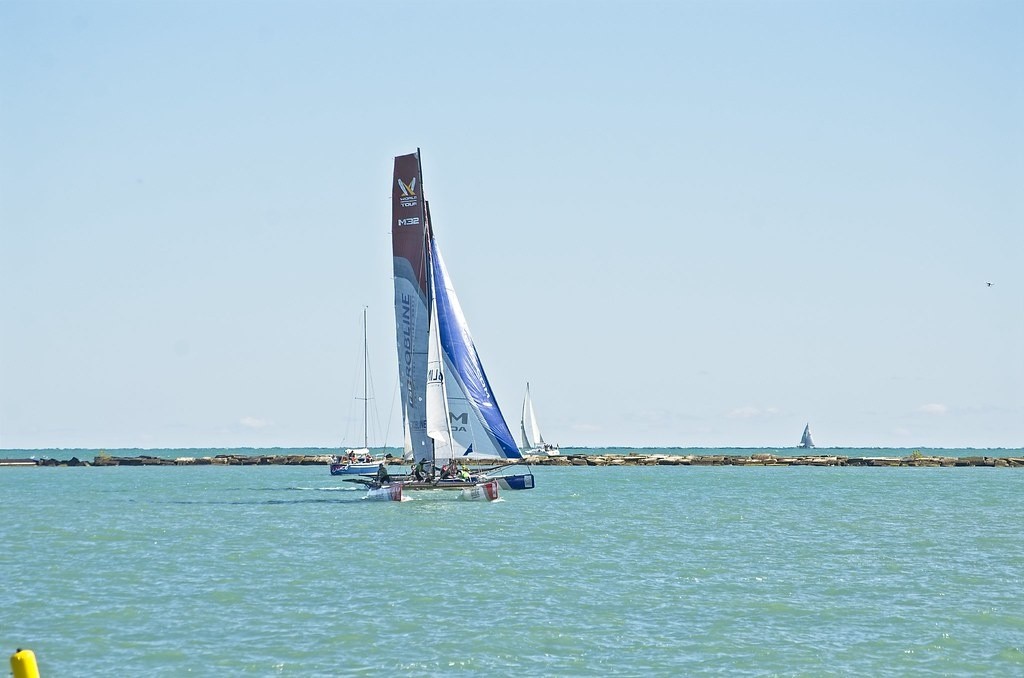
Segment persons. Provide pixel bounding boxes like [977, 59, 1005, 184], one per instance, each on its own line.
[415, 458, 431, 482]
[377, 463, 389, 483]
[349, 450, 355, 464]
[440, 464, 450, 479]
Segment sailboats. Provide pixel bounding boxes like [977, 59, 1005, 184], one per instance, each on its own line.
[329, 305, 387, 476]
[520, 381, 560, 457]
[342, 146, 534, 503]
[797, 422, 815, 450]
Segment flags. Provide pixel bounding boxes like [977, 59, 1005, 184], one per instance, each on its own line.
[463, 443, 473, 456]
